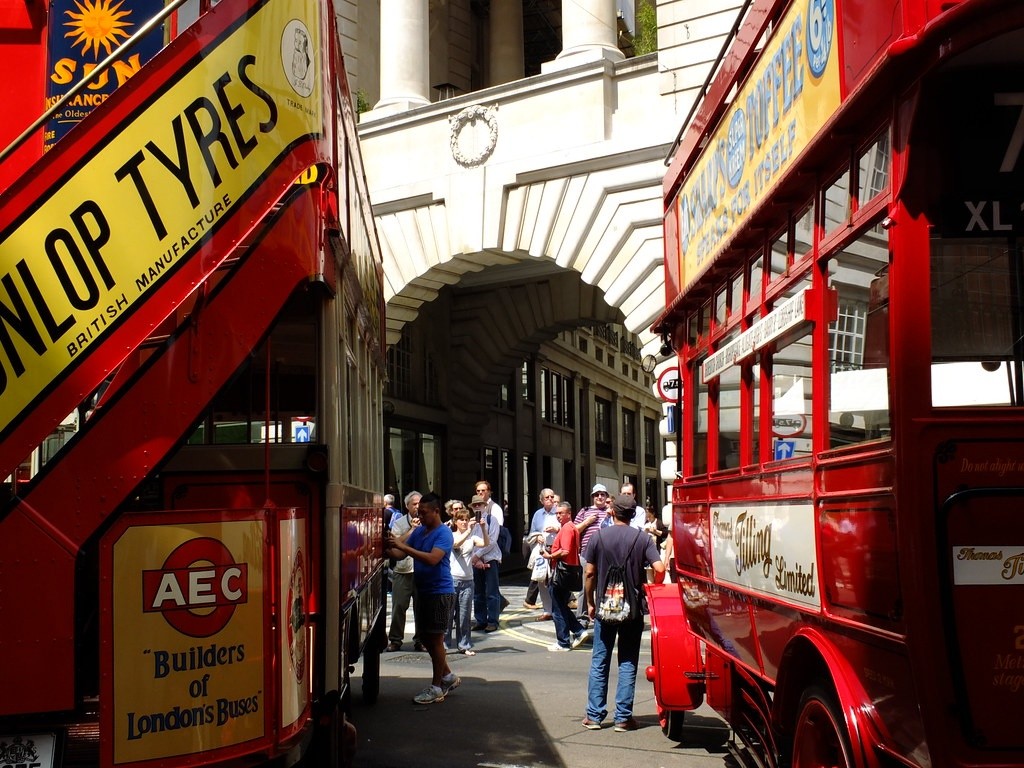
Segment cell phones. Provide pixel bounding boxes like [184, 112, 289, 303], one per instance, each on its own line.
[476, 511, 482, 522]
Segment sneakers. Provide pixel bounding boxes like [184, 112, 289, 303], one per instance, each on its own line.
[387, 642, 400, 651]
[581, 716, 602, 730]
[547, 642, 571, 652]
[570, 631, 590, 649]
[415, 643, 428, 652]
[413, 686, 444, 704]
[615, 714, 640, 732]
[437, 672, 461, 696]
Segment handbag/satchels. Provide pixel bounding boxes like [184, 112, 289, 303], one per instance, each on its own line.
[553, 555, 583, 591]
[531, 555, 554, 588]
[527, 538, 551, 570]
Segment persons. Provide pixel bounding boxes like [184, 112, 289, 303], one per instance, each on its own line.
[442, 480, 511, 656]
[387, 490, 428, 651]
[384, 492, 462, 704]
[582, 494, 665, 732]
[524, 484, 678, 652]
[383, 494, 400, 594]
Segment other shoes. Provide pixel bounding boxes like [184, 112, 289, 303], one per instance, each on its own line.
[484, 624, 496, 633]
[500, 596, 510, 614]
[471, 623, 487, 631]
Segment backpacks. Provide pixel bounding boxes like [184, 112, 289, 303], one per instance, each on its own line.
[595, 528, 643, 625]
[387, 508, 403, 530]
[487, 515, 512, 558]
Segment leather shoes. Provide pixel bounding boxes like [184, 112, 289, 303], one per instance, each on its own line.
[523, 601, 539, 608]
[567, 601, 578, 609]
[535, 614, 553, 621]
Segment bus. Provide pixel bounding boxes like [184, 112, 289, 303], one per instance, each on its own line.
[0, 0, 391, 767]
[639, 0, 1024, 768]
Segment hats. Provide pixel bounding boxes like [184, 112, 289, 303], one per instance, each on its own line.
[590, 484, 608, 496]
[468, 495, 488, 508]
[613, 494, 637, 518]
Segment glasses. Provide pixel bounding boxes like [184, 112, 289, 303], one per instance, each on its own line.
[594, 493, 605, 497]
[623, 493, 631, 496]
[605, 501, 612, 504]
[454, 508, 463, 511]
[472, 503, 482, 508]
